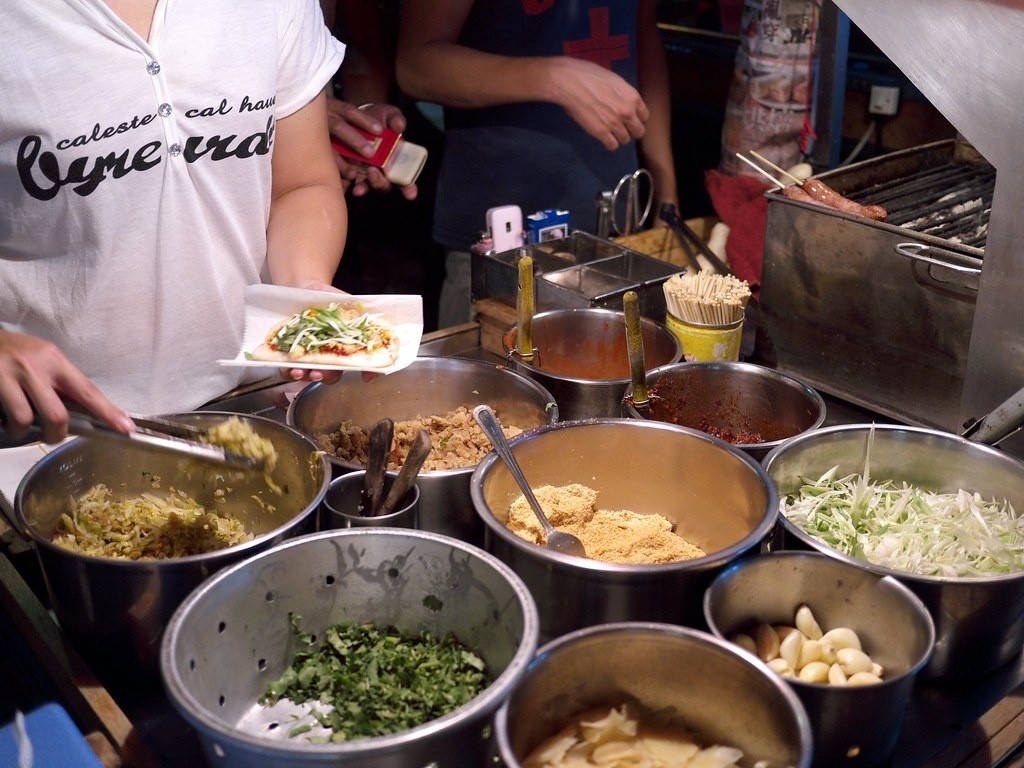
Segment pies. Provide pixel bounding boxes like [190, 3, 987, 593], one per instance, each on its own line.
[252, 305, 400, 368]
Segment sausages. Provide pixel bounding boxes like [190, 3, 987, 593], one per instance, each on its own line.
[804, 177, 887, 222]
[782, 185, 875, 221]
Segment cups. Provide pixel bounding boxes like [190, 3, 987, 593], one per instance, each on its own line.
[665, 310, 745, 363]
[320, 470, 421, 530]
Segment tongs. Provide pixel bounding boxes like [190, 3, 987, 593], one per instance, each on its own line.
[30, 401, 266, 475]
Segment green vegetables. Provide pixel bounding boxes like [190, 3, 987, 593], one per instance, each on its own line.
[256, 610, 492, 744]
[270, 302, 387, 352]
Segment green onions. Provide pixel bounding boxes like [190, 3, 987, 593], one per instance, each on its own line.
[778, 465, 1024, 579]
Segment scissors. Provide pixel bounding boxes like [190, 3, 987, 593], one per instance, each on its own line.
[610, 168, 655, 236]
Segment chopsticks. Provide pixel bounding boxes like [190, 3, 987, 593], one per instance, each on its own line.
[664, 269, 752, 324]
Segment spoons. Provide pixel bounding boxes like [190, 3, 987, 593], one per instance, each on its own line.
[473, 404, 585, 559]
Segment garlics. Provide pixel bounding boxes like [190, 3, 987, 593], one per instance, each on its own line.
[733, 603, 885, 686]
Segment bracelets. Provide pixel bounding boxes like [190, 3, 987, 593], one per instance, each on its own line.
[358, 103, 375, 110]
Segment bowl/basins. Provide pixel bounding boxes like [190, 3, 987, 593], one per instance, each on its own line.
[161, 526, 539, 768]
[620, 360, 826, 463]
[704, 550, 936, 768]
[287, 355, 559, 555]
[495, 620, 813, 768]
[760, 423, 1024, 692]
[479, 418, 780, 637]
[15, 409, 333, 684]
[502, 307, 683, 422]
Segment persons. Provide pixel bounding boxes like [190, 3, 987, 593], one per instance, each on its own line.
[398, 0, 682, 329]
[320, 0, 418, 201]
[0, 0, 350, 444]
